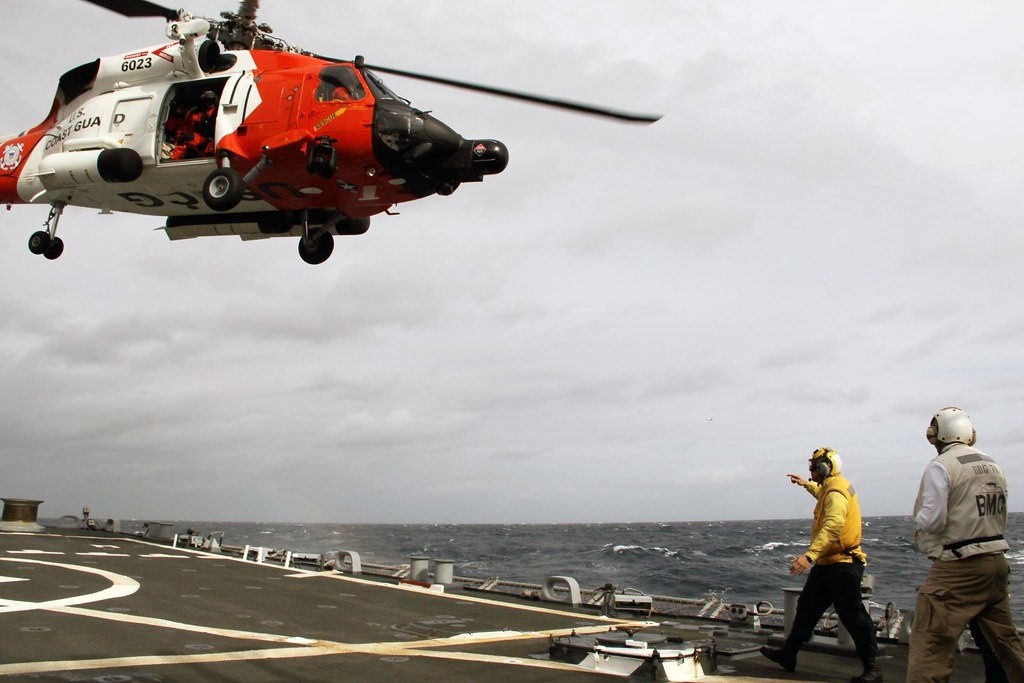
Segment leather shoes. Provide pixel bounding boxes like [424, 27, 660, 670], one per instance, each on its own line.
[760, 645, 797, 673]
[850, 659, 884, 683]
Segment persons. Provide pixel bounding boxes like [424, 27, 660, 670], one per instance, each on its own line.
[907, 406, 1024, 683]
[332, 80, 358, 99]
[759, 448, 883, 683]
[168, 90, 220, 158]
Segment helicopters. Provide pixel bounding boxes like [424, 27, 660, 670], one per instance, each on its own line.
[0, 0, 666, 264]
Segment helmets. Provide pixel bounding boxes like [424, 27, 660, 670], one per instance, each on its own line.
[809, 446, 842, 476]
[200, 91, 217, 102]
[927, 407, 976, 444]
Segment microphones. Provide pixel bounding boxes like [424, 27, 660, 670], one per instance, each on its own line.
[808, 478, 812, 481]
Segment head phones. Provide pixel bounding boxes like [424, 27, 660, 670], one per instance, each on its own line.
[926, 426, 976, 447]
[816, 450, 831, 477]
